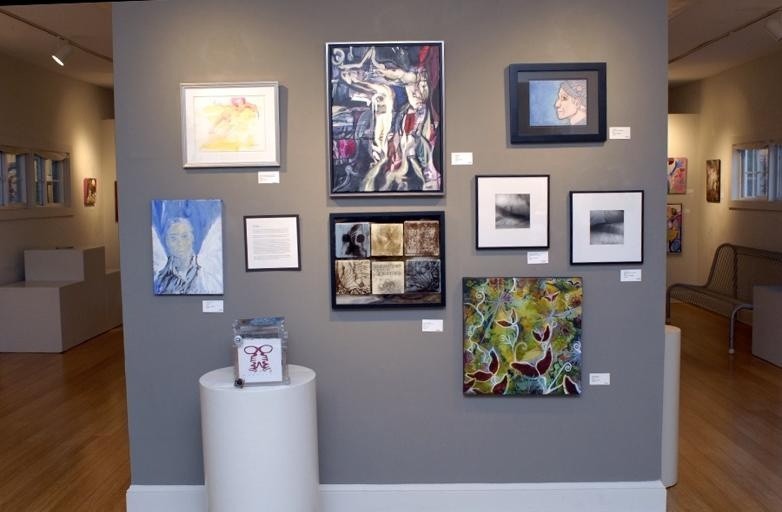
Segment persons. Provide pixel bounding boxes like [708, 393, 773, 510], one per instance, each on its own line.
[554, 78, 587, 125]
[154, 216, 222, 294]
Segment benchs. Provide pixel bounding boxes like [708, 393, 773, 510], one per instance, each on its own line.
[667, 245, 780, 354]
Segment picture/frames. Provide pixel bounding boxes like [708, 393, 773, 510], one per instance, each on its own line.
[476, 175, 550, 251]
[508, 61, 606, 141]
[328, 210, 447, 312]
[667, 204, 683, 253]
[570, 190, 644, 266]
[325, 39, 447, 200]
[180, 81, 281, 168]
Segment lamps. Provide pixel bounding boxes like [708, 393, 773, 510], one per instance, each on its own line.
[51, 41, 76, 66]
